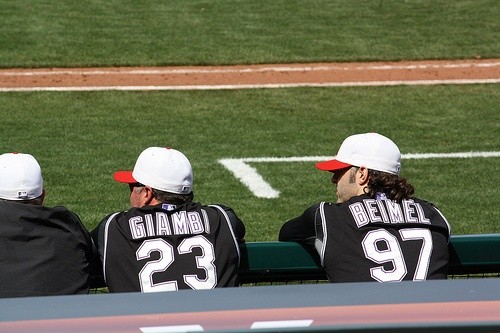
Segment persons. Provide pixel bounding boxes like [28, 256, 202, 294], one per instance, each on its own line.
[279, 132, 452, 282]
[0, 152, 92, 298]
[86, 145, 247, 293]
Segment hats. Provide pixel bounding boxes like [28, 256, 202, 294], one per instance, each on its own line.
[0, 152, 43, 200]
[114, 147, 193, 194]
[315, 132, 400, 176]
